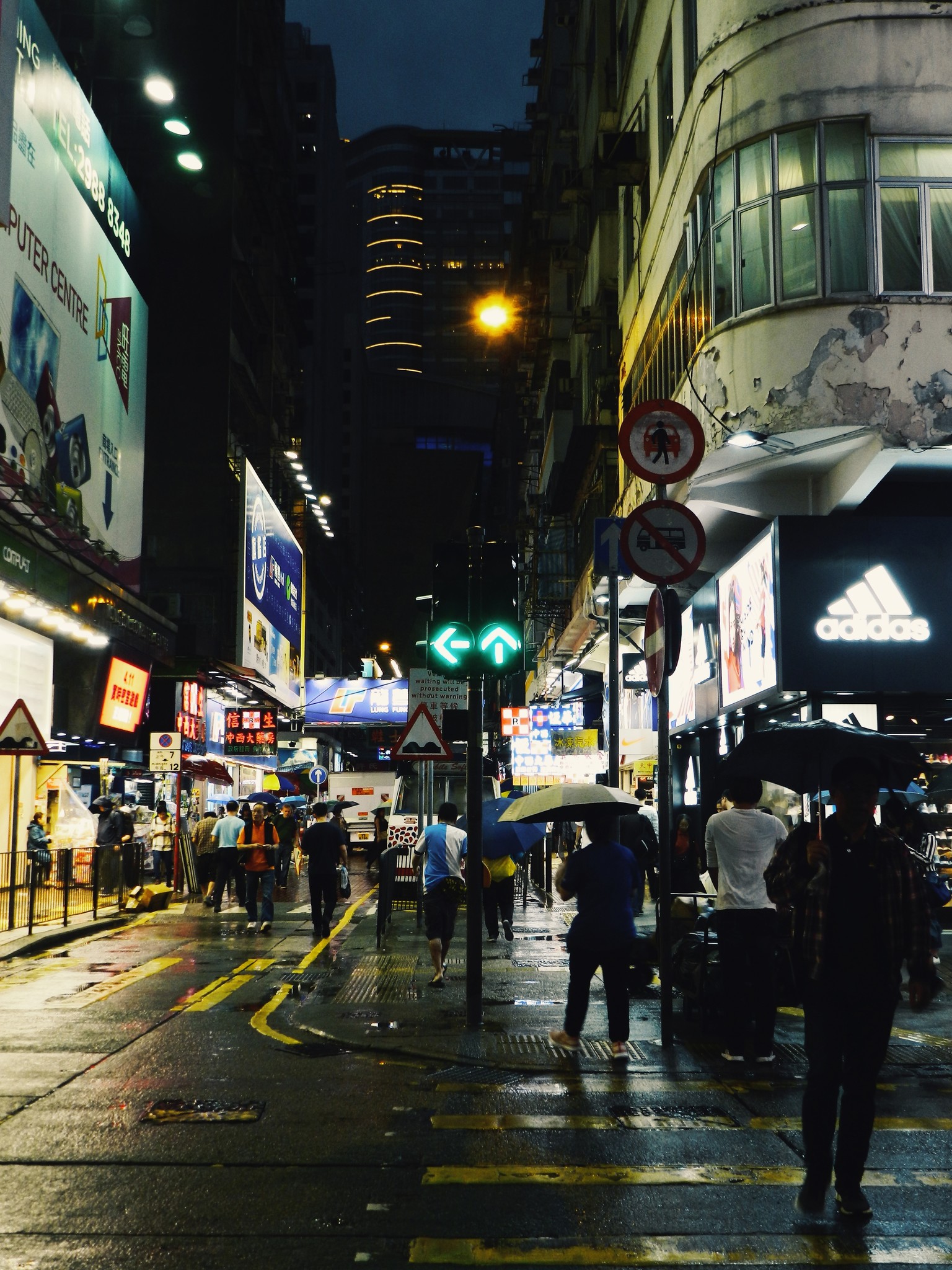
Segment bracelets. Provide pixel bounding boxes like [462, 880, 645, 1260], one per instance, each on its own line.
[271, 845, 274, 851]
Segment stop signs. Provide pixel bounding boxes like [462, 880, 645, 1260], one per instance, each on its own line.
[643, 587, 682, 699]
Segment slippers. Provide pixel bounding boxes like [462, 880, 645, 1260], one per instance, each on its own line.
[435, 963, 447, 976]
[428, 978, 446, 988]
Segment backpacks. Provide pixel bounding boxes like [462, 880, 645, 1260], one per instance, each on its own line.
[483, 854, 516, 883]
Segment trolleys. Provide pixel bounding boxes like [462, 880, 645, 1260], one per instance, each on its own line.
[654, 889, 732, 1036]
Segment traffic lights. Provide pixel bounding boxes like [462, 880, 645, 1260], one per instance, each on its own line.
[426, 620, 477, 673]
[478, 621, 523, 673]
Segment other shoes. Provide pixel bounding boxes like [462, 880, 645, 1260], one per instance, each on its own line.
[312, 930, 321, 936]
[247, 921, 256, 929]
[548, 1030, 581, 1051]
[755, 1046, 776, 1063]
[322, 917, 330, 938]
[203, 896, 213, 906]
[611, 1041, 628, 1058]
[793, 1181, 825, 1214]
[721, 1043, 744, 1061]
[502, 919, 514, 942]
[275, 877, 287, 888]
[485, 936, 497, 943]
[260, 921, 272, 933]
[835, 1182, 872, 1215]
[99, 888, 116, 896]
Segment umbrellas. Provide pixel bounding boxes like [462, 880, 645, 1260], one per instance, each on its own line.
[455, 782, 643, 860]
[293, 839, 303, 880]
[370, 799, 392, 816]
[206, 792, 307, 809]
[182, 754, 234, 820]
[715, 718, 952, 841]
[262, 773, 296, 794]
[327, 801, 360, 813]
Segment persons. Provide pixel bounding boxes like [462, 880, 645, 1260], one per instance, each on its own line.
[191, 800, 296, 912]
[548, 821, 592, 861]
[236, 803, 280, 933]
[552, 812, 639, 1058]
[614, 788, 734, 918]
[330, 810, 347, 866]
[301, 803, 347, 938]
[366, 807, 388, 870]
[412, 802, 468, 988]
[192, 790, 200, 796]
[686, 777, 788, 1062]
[92, 796, 139, 897]
[481, 855, 517, 942]
[765, 758, 937, 1217]
[26, 812, 52, 886]
[150, 800, 176, 886]
[885, 797, 941, 966]
[194, 797, 200, 806]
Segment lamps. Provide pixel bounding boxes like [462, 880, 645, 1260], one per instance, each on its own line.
[564, 655, 577, 666]
[535, 666, 562, 699]
[219, 677, 291, 722]
[254, 446, 335, 540]
[54, 8, 153, 47]
[595, 594, 609, 603]
[0, 482, 120, 575]
[720, 430, 797, 453]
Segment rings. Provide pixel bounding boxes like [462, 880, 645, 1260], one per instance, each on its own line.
[267, 849, 268, 850]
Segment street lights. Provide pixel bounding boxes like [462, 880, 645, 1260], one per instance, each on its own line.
[465, 289, 627, 785]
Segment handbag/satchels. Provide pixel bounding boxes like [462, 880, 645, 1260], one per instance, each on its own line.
[921, 855, 950, 906]
